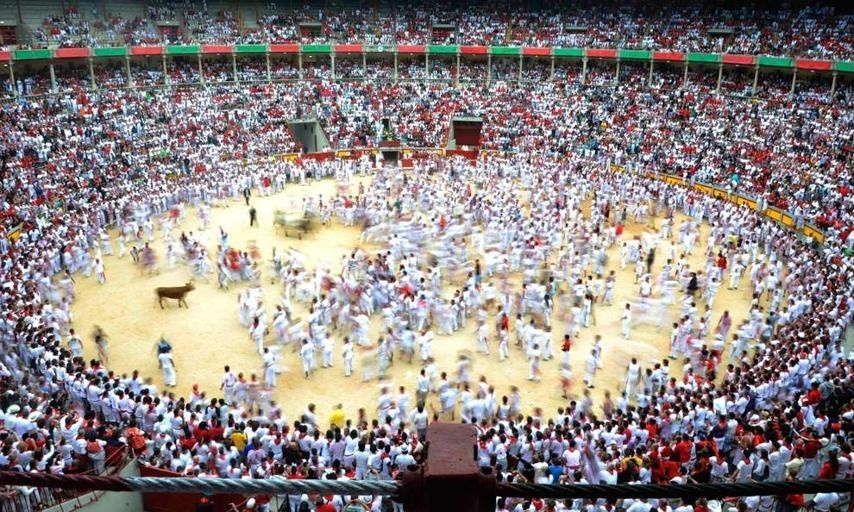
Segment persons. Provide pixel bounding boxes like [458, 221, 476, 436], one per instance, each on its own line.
[1, 0, 854, 511]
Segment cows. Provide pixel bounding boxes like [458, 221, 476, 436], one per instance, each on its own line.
[153, 280, 196, 308]
